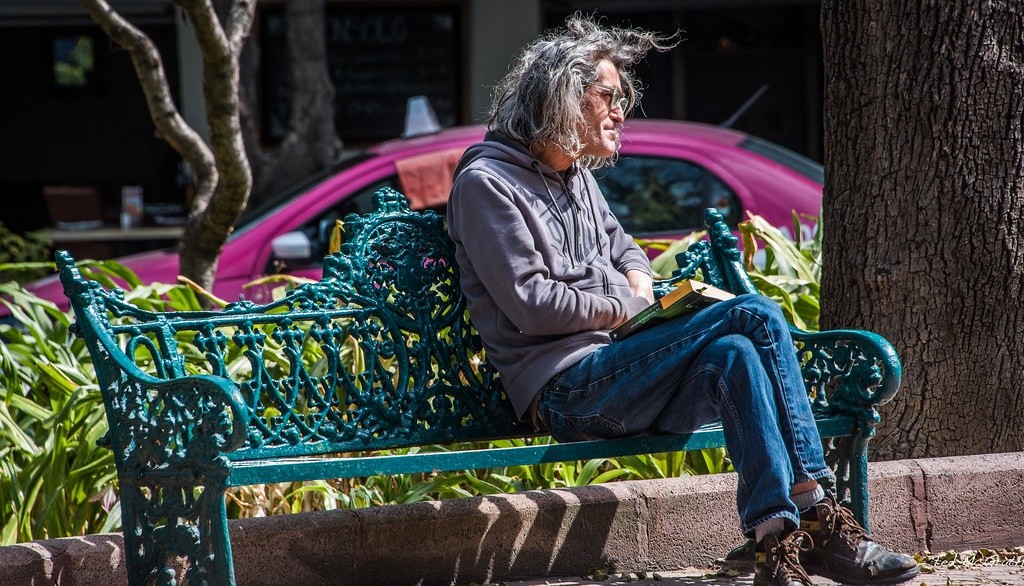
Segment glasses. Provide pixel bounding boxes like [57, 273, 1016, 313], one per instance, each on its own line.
[582, 79, 629, 114]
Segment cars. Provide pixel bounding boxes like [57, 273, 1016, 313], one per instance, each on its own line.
[1, 124, 825, 334]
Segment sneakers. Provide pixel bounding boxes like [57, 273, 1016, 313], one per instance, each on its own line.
[753, 527, 815, 586]
[795, 489, 919, 586]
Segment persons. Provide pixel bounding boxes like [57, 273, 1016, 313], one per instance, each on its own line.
[446, 12, 919, 586]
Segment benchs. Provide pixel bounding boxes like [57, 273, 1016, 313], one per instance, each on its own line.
[54, 185, 903, 586]
